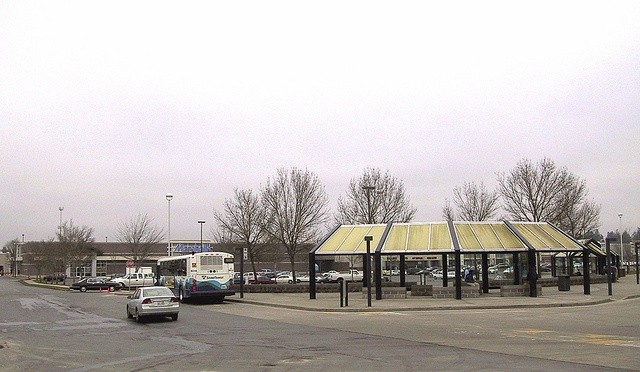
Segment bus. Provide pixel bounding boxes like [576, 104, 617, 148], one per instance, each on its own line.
[157, 252, 235, 303]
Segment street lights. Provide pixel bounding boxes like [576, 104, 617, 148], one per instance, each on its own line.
[59, 207, 64, 241]
[166, 194, 173, 257]
[361, 186, 375, 224]
[618, 212, 625, 267]
[198, 220, 205, 252]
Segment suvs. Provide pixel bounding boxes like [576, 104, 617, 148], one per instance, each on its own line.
[540, 262, 551, 272]
[571, 262, 582, 275]
[492, 263, 509, 268]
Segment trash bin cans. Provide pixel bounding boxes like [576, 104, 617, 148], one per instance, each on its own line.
[556, 275, 570, 291]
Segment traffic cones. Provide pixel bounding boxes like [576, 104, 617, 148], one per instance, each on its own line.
[107, 288, 111, 294]
[98, 287, 103, 294]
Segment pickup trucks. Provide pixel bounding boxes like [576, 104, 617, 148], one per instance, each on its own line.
[110, 273, 155, 289]
[330, 269, 364, 284]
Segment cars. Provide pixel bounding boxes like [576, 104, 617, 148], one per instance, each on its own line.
[243, 271, 264, 283]
[408, 266, 455, 279]
[314, 270, 340, 284]
[127, 286, 179, 321]
[272, 271, 290, 275]
[261, 269, 271, 274]
[233, 272, 241, 284]
[271, 275, 295, 284]
[462, 265, 497, 274]
[296, 274, 309, 283]
[381, 269, 406, 275]
[504, 265, 514, 274]
[72, 277, 121, 292]
